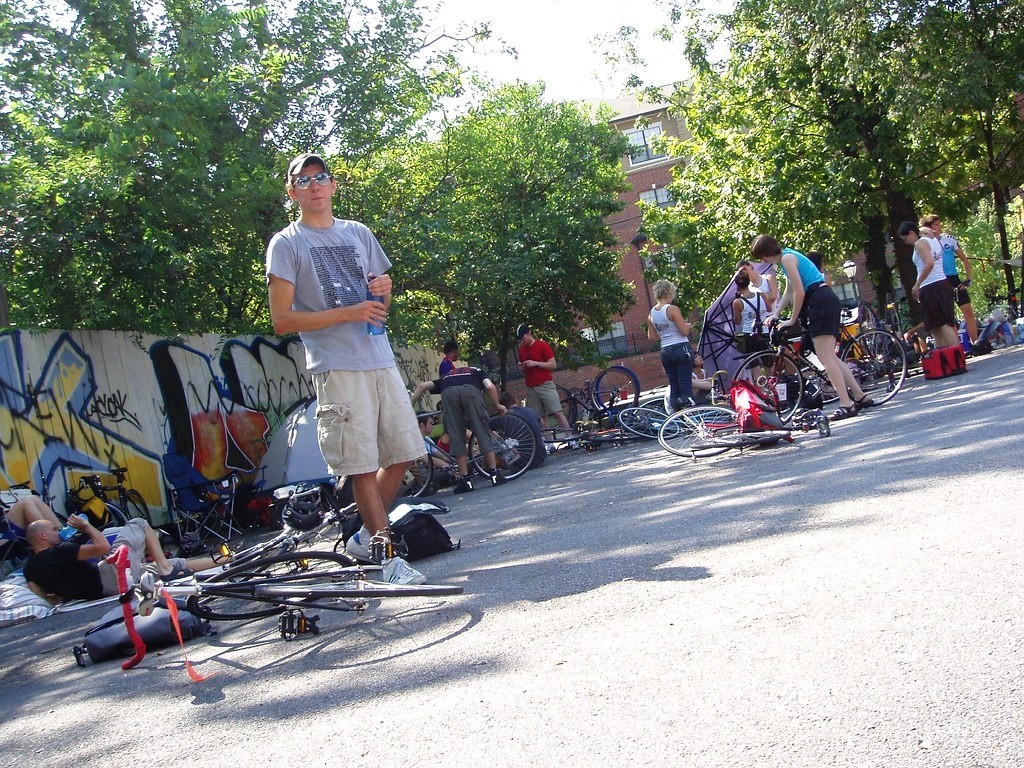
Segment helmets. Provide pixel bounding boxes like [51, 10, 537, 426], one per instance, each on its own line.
[180, 532, 203, 552]
[282, 504, 327, 530]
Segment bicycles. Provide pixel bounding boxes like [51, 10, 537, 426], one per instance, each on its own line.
[731, 312, 907, 426]
[393, 389, 538, 498]
[68, 467, 152, 540]
[105, 480, 466, 671]
[982, 288, 1023, 324]
[539, 298, 938, 464]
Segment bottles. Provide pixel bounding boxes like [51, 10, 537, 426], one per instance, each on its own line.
[366, 277, 385, 335]
[58, 514, 89, 542]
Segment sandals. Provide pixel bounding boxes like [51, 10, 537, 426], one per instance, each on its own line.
[858, 395, 875, 410]
[826, 403, 857, 420]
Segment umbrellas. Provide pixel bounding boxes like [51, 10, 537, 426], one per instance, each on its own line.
[696, 262, 780, 395]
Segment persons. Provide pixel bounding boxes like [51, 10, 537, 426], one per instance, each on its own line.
[733, 260, 782, 410]
[438, 340, 458, 378]
[408, 365, 509, 493]
[435, 397, 520, 469]
[690, 349, 719, 406]
[646, 278, 701, 438]
[897, 220, 963, 369]
[0, 493, 76, 540]
[265, 153, 432, 585]
[752, 234, 874, 421]
[516, 323, 580, 452]
[414, 411, 476, 482]
[806, 250, 833, 288]
[918, 215, 992, 356]
[22, 517, 242, 606]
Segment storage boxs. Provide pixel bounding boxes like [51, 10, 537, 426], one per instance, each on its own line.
[929, 329, 971, 350]
[921, 344, 967, 379]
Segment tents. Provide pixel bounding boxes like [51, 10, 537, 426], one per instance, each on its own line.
[252, 394, 353, 495]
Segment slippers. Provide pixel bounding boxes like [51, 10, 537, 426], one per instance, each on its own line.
[160, 565, 194, 583]
[230, 541, 243, 553]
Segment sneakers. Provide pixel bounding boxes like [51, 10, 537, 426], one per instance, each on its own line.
[345, 531, 377, 562]
[491, 474, 507, 486]
[382, 558, 427, 586]
[453, 476, 473, 494]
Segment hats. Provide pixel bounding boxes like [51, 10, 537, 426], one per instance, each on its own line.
[287, 152, 329, 183]
[519, 323, 530, 339]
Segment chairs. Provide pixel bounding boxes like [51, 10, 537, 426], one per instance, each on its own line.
[162, 454, 267, 542]
[0, 480, 68, 577]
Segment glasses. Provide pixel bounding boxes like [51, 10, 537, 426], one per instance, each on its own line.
[290, 172, 331, 189]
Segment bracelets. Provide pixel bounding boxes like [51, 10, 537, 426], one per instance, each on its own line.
[963, 278, 973, 287]
[536, 361, 539, 366]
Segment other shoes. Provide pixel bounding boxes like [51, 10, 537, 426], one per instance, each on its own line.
[569, 438, 580, 450]
[973, 342, 990, 355]
[550, 444, 558, 453]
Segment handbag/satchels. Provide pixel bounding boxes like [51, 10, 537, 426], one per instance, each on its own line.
[84, 600, 211, 663]
[783, 376, 824, 410]
[921, 344, 966, 379]
[733, 331, 757, 353]
[393, 516, 457, 563]
